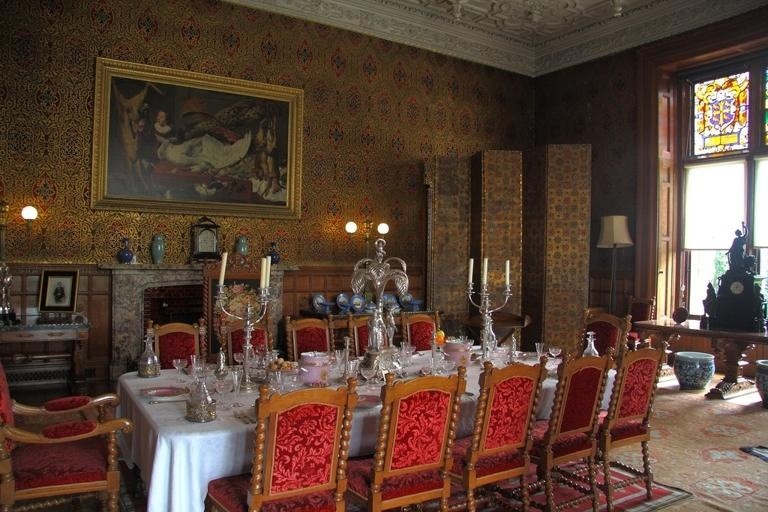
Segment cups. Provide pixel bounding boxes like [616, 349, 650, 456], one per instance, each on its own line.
[172, 335, 563, 400]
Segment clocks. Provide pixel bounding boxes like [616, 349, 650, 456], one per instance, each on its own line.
[700, 221, 765, 333]
[192, 215, 220, 258]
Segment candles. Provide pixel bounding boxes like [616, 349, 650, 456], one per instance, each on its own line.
[505, 260, 510, 284]
[483, 258, 488, 284]
[469, 258, 474, 283]
[219, 252, 228, 285]
[265, 255, 272, 288]
[260, 257, 266, 289]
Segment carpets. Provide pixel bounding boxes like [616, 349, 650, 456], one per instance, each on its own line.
[410, 456, 691, 512]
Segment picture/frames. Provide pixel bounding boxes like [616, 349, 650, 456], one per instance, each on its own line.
[37, 267, 80, 313]
[90, 55, 305, 220]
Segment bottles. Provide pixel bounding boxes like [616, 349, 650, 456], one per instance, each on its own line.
[185, 368, 217, 424]
[582, 332, 600, 358]
[138, 329, 161, 378]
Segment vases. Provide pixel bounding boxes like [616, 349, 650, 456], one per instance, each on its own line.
[117, 237, 133, 264]
[674, 351, 716, 390]
[268, 242, 279, 262]
[755, 360, 768, 402]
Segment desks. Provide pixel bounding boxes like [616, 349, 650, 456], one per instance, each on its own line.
[634, 318, 768, 378]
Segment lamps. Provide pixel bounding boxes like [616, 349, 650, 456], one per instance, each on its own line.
[344, 219, 390, 258]
[21, 205, 38, 231]
[596, 214, 635, 314]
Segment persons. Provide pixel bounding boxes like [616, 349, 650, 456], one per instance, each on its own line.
[53, 281, 66, 303]
[139, 108, 175, 161]
[725, 220, 748, 272]
